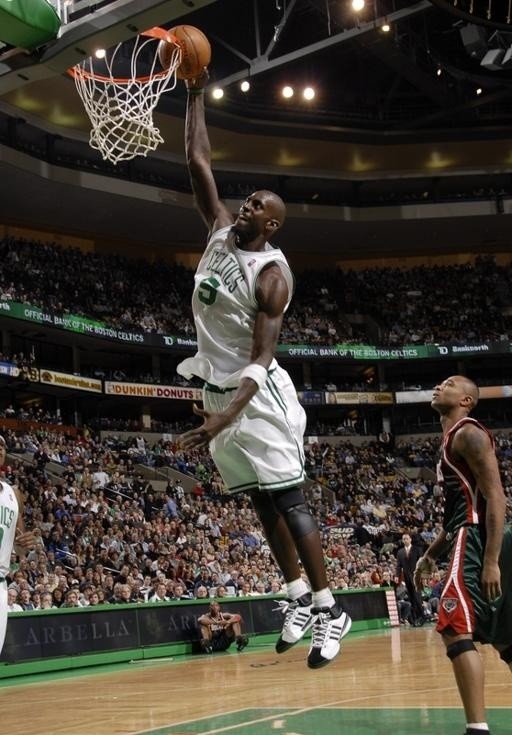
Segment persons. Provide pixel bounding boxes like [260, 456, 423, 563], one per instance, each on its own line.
[396, 571, 442, 623]
[1, 436, 37, 648]
[1, 225, 511, 392]
[197, 600, 249, 654]
[393, 532, 430, 622]
[1, 114, 512, 205]
[175, 65, 353, 668]
[0, 400, 512, 613]
[412, 374, 511, 735]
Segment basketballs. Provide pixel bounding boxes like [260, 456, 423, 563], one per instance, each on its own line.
[158, 25, 211, 80]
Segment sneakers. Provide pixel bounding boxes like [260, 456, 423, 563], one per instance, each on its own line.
[200, 638, 213, 654]
[237, 637, 249, 651]
[275, 591, 352, 670]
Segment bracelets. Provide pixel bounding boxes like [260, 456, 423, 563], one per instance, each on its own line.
[187, 86, 204, 94]
